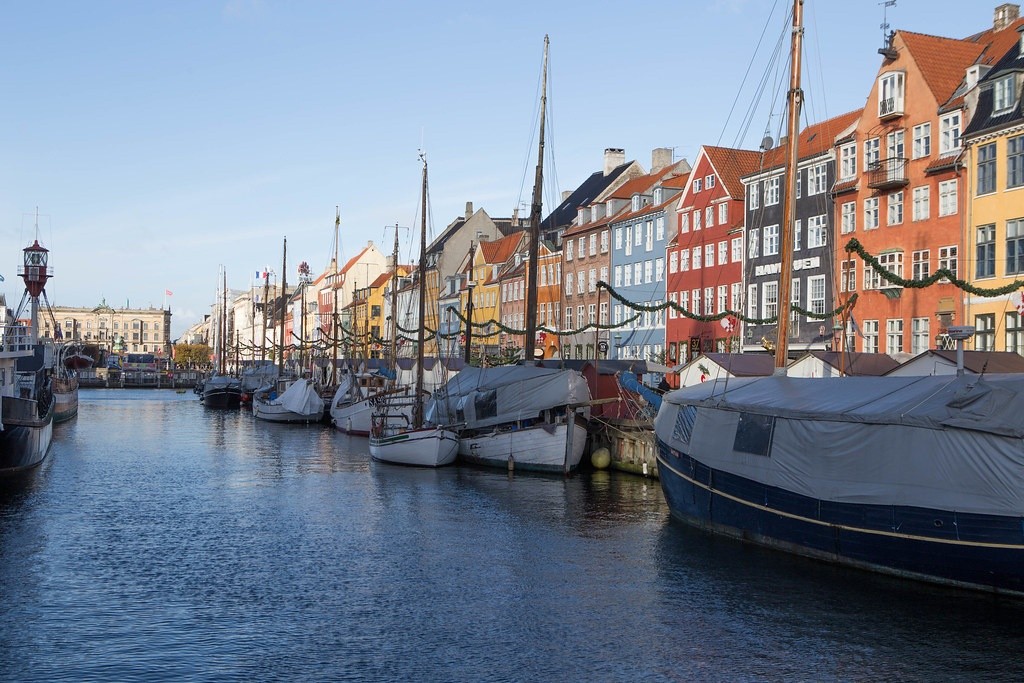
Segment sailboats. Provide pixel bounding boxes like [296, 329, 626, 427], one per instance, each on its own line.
[193, 33, 593, 477]
[652, 0, 1024, 601]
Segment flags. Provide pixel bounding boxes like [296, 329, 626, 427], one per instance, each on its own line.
[255, 271, 267, 278]
[166, 290, 173, 298]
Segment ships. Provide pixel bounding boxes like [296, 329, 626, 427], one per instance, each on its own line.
[0, 293, 57, 476]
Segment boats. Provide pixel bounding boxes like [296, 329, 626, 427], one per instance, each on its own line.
[50, 372, 78, 424]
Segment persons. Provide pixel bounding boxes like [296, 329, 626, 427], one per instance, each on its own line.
[657, 377, 671, 391]
[884, 30, 897, 49]
